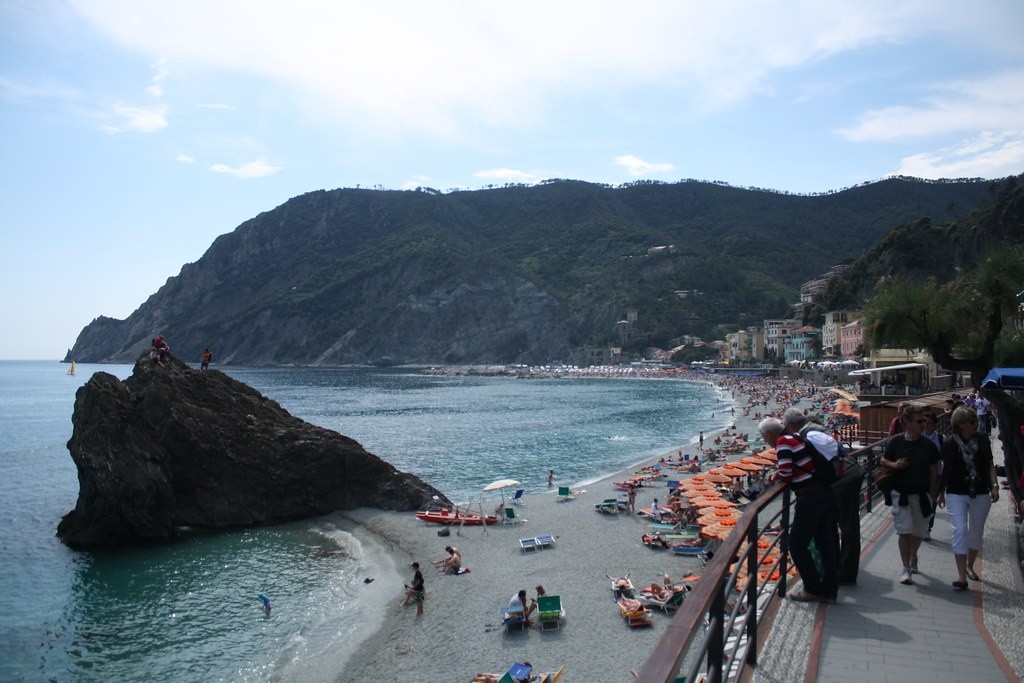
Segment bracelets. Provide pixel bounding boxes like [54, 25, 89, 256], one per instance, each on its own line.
[990, 482, 999, 489]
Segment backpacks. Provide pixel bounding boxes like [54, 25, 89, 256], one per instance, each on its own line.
[787, 430, 846, 485]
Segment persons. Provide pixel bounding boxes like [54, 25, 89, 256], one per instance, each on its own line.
[505, 589, 534, 627]
[154, 335, 171, 353]
[604, 572, 633, 591]
[620, 592, 644, 614]
[696, 431, 705, 450]
[936, 405, 1000, 591]
[525, 585, 551, 618]
[149, 346, 166, 369]
[393, 357, 1024, 683]
[880, 405, 939, 584]
[626, 484, 637, 516]
[410, 561, 427, 616]
[200, 348, 212, 376]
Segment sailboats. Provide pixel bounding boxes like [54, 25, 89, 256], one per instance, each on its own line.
[66, 360, 77, 375]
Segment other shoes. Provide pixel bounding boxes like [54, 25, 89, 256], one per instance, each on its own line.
[965, 566, 979, 580]
[953, 580, 969, 589]
[790, 590, 837, 604]
[922, 531, 931, 541]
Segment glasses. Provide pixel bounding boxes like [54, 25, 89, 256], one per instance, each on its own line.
[961, 419, 979, 426]
[909, 418, 926, 424]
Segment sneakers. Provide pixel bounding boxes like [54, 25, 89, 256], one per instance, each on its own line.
[911, 558, 918, 574]
[900, 566, 912, 584]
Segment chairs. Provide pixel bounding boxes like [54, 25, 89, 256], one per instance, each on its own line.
[499, 442, 773, 683]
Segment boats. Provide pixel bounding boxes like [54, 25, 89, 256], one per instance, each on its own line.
[415, 511, 498, 525]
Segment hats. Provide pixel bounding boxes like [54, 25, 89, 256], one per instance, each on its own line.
[923, 412, 939, 425]
[945, 399, 954, 405]
